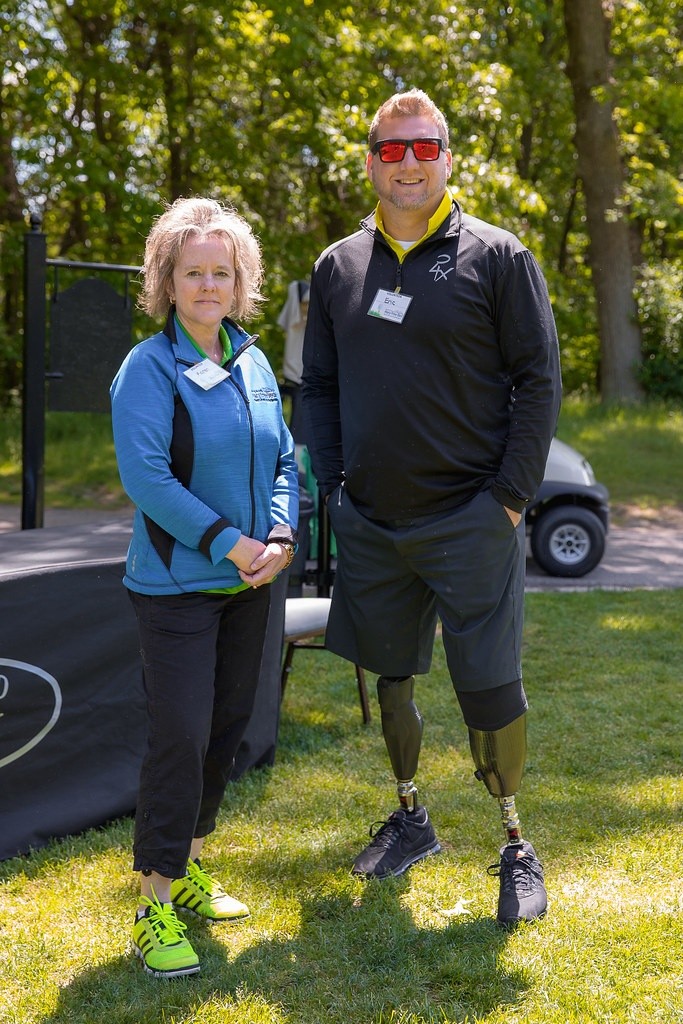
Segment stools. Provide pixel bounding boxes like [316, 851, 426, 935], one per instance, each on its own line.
[283, 595, 373, 726]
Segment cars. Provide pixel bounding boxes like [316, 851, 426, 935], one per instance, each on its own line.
[522, 435, 614, 575]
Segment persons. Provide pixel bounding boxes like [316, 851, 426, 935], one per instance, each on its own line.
[107, 188, 306, 976]
[304, 86, 562, 926]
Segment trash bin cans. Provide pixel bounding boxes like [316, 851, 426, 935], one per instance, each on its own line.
[285, 483, 316, 599]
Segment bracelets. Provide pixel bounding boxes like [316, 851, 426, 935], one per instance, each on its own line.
[280, 542, 295, 570]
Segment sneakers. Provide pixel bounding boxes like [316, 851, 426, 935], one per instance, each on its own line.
[130, 881, 202, 978]
[353, 806, 440, 880]
[170, 856, 252, 925]
[487, 839, 547, 926]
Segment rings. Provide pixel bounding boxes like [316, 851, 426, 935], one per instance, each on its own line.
[252, 585, 259, 590]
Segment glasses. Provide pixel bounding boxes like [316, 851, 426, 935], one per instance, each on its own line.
[371, 138, 445, 164]
